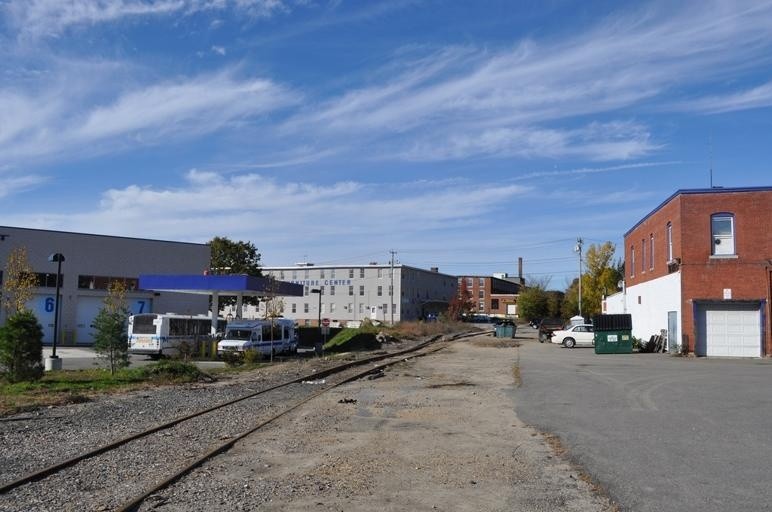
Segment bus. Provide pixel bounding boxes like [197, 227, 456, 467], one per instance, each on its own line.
[126, 313, 227, 360]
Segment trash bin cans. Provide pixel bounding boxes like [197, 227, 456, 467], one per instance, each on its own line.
[496, 321, 516, 338]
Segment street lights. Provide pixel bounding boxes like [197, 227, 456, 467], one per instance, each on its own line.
[45, 253, 65, 370]
[310, 289, 322, 327]
[575, 236, 583, 315]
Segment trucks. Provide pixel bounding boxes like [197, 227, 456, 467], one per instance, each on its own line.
[217, 318, 294, 362]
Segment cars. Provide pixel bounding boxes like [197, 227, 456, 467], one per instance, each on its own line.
[426, 313, 490, 323]
[530, 318, 595, 348]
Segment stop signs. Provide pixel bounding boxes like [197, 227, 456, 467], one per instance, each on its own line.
[322, 318, 330, 327]
